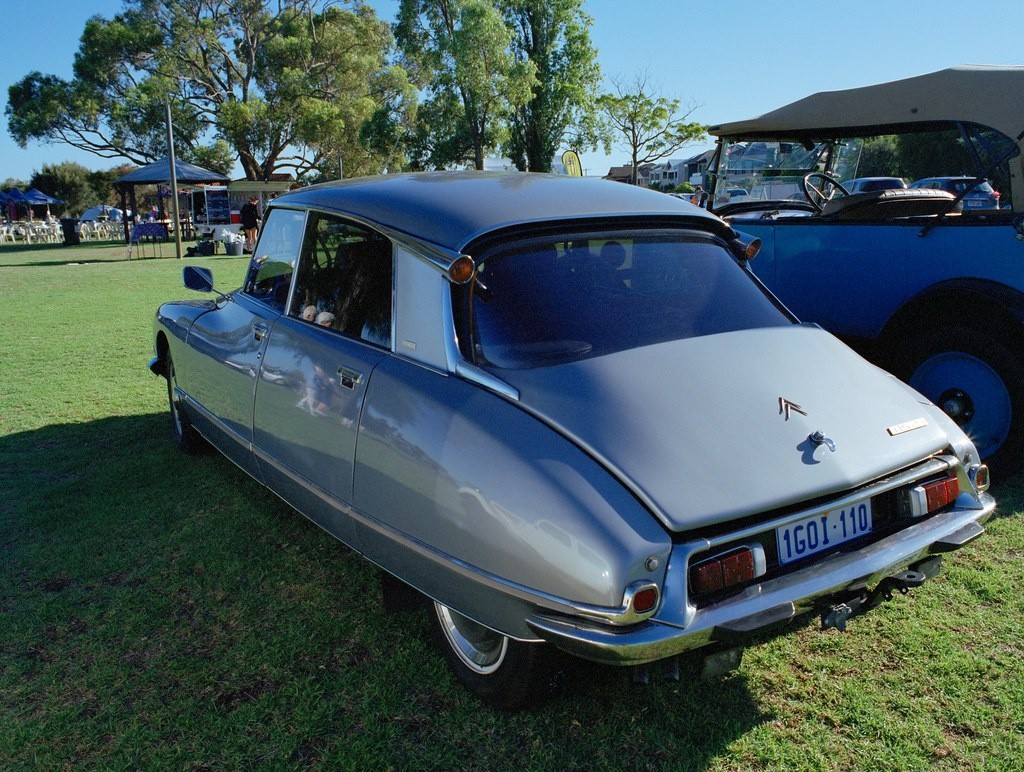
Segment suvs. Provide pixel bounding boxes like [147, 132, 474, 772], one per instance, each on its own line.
[725, 189, 750, 203]
[907, 175, 1000, 212]
[562, 67, 1024, 476]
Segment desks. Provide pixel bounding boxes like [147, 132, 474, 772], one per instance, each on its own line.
[34, 224, 63, 243]
[130, 222, 169, 243]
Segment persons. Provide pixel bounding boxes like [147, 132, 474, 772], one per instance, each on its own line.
[143, 204, 158, 221]
[695, 186, 706, 207]
[164, 212, 169, 218]
[246, 256, 268, 292]
[300, 305, 335, 417]
[179, 209, 190, 230]
[109, 199, 131, 220]
[240, 196, 261, 252]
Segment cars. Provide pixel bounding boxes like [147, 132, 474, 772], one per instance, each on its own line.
[148, 169, 998, 711]
[785, 191, 815, 203]
[677, 193, 700, 205]
[833, 177, 907, 196]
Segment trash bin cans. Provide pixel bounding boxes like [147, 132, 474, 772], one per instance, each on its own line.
[198, 241, 215, 256]
[60, 217, 80, 245]
[224, 241, 243, 256]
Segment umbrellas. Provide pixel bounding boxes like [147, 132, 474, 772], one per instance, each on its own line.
[0, 188, 65, 221]
[112, 154, 228, 216]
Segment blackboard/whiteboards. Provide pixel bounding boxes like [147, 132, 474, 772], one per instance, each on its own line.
[204, 188, 231, 225]
[191, 190, 207, 226]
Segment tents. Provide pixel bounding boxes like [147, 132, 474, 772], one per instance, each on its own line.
[80, 205, 113, 228]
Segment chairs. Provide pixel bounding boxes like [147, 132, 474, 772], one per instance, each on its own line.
[0, 219, 175, 245]
